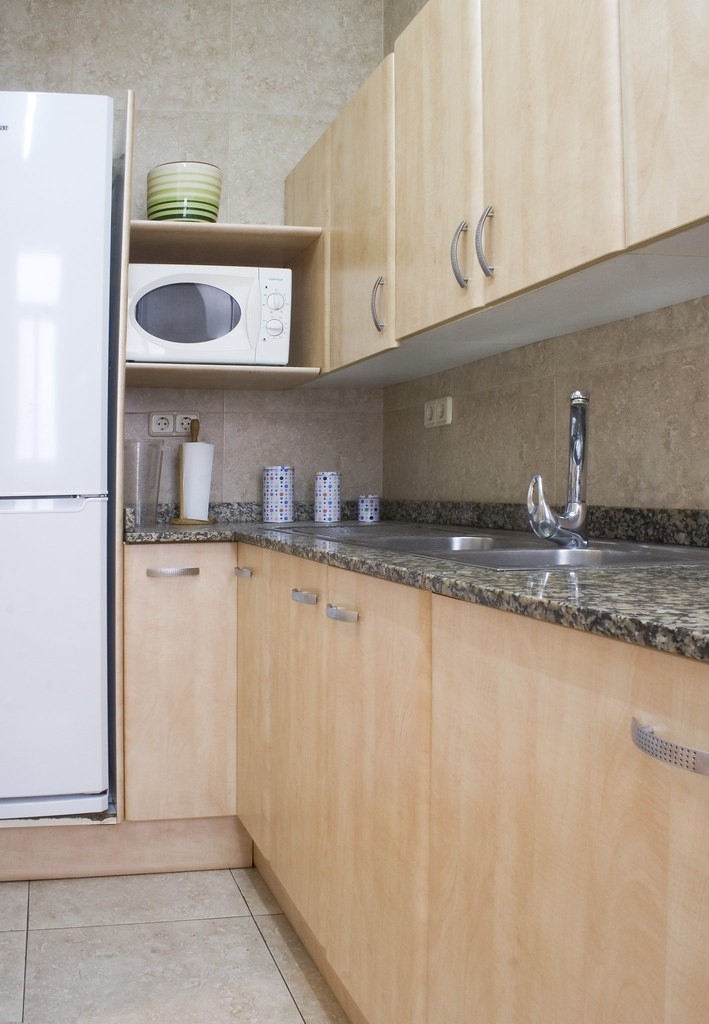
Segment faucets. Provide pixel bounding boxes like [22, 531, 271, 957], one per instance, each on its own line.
[527, 389, 590, 550]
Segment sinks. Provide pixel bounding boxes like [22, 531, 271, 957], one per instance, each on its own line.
[403, 550, 709, 570]
[330, 536, 593, 552]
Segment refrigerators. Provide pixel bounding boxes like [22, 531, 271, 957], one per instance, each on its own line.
[0, 91, 117, 821]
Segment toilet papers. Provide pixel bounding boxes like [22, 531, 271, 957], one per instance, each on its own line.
[179, 441, 215, 522]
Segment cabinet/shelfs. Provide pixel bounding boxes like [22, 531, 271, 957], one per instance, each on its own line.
[118, 542, 709, 1024]
[124, 0, 709, 396]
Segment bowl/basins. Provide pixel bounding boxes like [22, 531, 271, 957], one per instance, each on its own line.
[146, 159, 223, 223]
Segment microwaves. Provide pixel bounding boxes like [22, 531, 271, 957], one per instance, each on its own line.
[126, 263, 293, 365]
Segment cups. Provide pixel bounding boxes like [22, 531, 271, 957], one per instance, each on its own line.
[358, 494, 380, 522]
[261, 466, 294, 523]
[314, 472, 342, 522]
[124, 438, 164, 533]
[178, 442, 214, 521]
